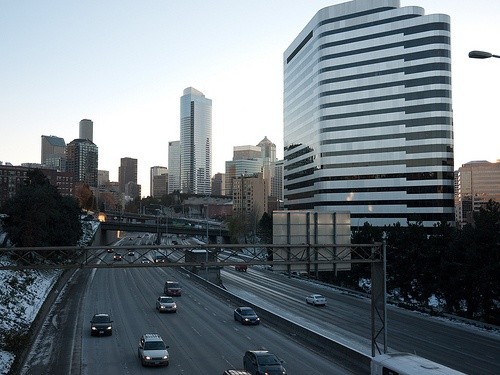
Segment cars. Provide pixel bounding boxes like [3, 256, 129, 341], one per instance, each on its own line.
[89, 313, 114, 337]
[163, 280, 183, 297]
[156, 296, 177, 313]
[106, 233, 187, 264]
[233, 307, 260, 326]
[235, 265, 247, 272]
[224, 369, 251, 375]
[305, 294, 328, 307]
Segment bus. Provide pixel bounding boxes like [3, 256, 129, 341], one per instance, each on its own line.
[370, 351, 468, 375]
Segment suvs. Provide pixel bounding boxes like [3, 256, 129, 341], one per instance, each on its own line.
[243, 348, 287, 375]
[137, 333, 170, 367]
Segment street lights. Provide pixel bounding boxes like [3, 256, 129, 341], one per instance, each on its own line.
[203, 204, 209, 272]
[155, 209, 168, 258]
[148, 203, 163, 226]
